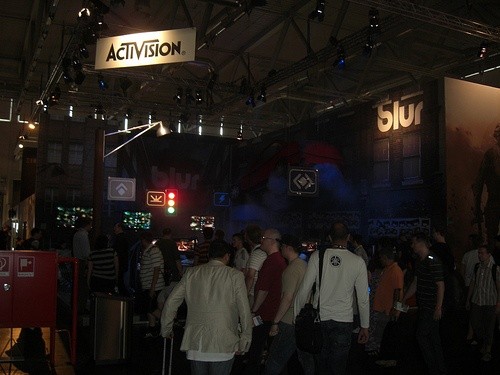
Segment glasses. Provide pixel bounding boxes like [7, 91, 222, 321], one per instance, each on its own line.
[259, 236, 278, 241]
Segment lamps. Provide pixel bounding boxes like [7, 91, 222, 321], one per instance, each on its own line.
[477, 39, 489, 59]
[309, 0, 326, 22]
[245, 92, 256, 108]
[236, 128, 243, 141]
[363, 34, 374, 54]
[257, 86, 267, 103]
[368, 7, 383, 35]
[332, 46, 346, 68]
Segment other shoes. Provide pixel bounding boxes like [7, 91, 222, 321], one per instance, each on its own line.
[243, 350, 266, 364]
[466, 330, 474, 339]
[375, 360, 398, 368]
[480, 347, 486, 353]
[481, 354, 491, 362]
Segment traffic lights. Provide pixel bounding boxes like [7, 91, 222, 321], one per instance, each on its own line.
[163, 188, 177, 217]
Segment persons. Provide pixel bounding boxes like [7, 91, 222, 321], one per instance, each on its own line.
[13, 217, 500, 375]
[472, 124, 500, 264]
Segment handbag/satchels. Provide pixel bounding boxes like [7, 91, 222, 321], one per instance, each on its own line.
[295, 303, 324, 354]
[379, 312, 405, 361]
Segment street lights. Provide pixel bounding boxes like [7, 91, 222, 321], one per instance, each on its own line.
[92, 121, 171, 249]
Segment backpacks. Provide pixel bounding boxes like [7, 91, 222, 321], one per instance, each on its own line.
[148, 246, 173, 287]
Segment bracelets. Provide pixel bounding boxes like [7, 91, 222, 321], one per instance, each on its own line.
[271, 322, 279, 325]
